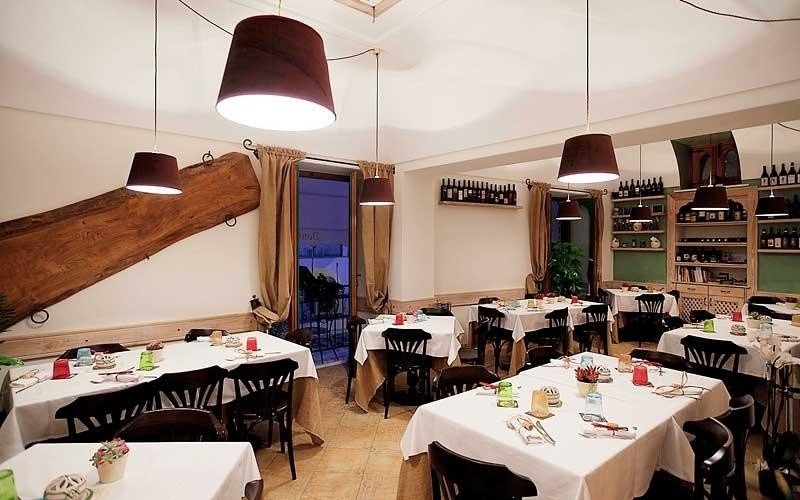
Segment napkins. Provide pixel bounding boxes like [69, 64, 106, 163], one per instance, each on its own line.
[510, 414, 545, 445]
[90, 367, 140, 382]
[8, 370, 51, 394]
[196, 336, 227, 342]
[650, 372, 713, 400]
[715, 313, 732, 320]
[550, 355, 576, 368]
[578, 420, 638, 440]
[367, 318, 384, 326]
[682, 323, 705, 329]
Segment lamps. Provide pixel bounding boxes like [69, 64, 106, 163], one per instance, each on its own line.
[752, 122, 790, 217]
[556, 183, 583, 221]
[628, 144, 653, 223]
[357, 47, 397, 208]
[215, 0, 338, 132]
[126, 0, 184, 197]
[690, 134, 731, 211]
[557, 0, 621, 185]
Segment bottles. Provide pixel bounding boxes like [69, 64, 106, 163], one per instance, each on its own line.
[612, 206, 654, 232]
[440, 178, 517, 206]
[760, 223, 799, 249]
[761, 162, 800, 186]
[619, 176, 663, 198]
[677, 199, 747, 222]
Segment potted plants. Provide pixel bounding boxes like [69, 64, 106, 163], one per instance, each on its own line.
[576, 365, 601, 398]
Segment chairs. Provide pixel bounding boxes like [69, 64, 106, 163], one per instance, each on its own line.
[537, 306, 569, 357]
[345, 315, 367, 404]
[597, 287, 610, 303]
[427, 440, 538, 500]
[183, 328, 231, 342]
[574, 304, 609, 355]
[679, 334, 749, 378]
[436, 365, 499, 400]
[635, 293, 666, 348]
[149, 365, 229, 424]
[747, 294, 786, 303]
[667, 289, 681, 304]
[54, 382, 150, 443]
[476, 296, 507, 374]
[381, 327, 432, 419]
[421, 307, 454, 316]
[523, 345, 565, 367]
[56, 343, 131, 360]
[227, 357, 299, 480]
[114, 407, 227, 441]
[630, 348, 757, 500]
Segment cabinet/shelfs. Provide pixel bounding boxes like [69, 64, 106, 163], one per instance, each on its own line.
[612, 194, 666, 253]
[667, 185, 758, 321]
[757, 183, 800, 254]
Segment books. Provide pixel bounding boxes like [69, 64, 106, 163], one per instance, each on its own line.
[676, 265, 747, 286]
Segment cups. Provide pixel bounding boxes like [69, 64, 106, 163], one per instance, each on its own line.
[492, 294, 585, 312]
[617, 353, 632, 372]
[584, 391, 604, 423]
[1, 469, 21, 500]
[632, 364, 650, 386]
[392, 309, 428, 326]
[620, 285, 653, 293]
[580, 355, 595, 368]
[622, 239, 646, 248]
[531, 389, 550, 418]
[496, 381, 517, 409]
[209, 330, 258, 350]
[704, 311, 774, 339]
[52, 347, 153, 379]
